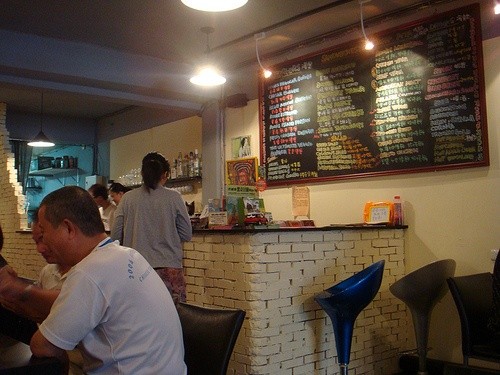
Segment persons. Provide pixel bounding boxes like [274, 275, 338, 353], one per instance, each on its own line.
[109, 183, 129, 205]
[0, 225, 21, 282]
[29, 186, 187, 375]
[88, 183, 118, 231]
[110, 152, 192, 304]
[0, 214, 85, 375]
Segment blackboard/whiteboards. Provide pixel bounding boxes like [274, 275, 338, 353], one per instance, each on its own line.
[256, 2, 491, 187]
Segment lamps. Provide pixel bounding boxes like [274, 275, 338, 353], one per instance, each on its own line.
[27, 90, 55, 148]
[180, 0, 375, 88]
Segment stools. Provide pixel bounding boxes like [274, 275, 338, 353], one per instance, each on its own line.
[314, 258, 456, 375]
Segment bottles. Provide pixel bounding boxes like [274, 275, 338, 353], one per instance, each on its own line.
[61, 156, 69, 169]
[183, 153, 188, 176]
[188, 151, 194, 177]
[55, 157, 62, 168]
[68, 156, 73, 169]
[393, 195, 403, 226]
[199, 154, 202, 177]
[177, 152, 183, 178]
[170, 159, 177, 179]
[193, 149, 199, 177]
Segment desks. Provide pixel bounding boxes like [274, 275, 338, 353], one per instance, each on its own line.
[0, 331, 70, 375]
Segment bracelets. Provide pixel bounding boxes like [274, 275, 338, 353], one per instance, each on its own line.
[20, 286, 33, 304]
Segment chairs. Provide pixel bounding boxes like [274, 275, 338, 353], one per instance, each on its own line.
[174, 302, 248, 375]
[444, 271, 500, 375]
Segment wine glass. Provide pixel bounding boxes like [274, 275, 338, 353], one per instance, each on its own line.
[118, 168, 142, 186]
